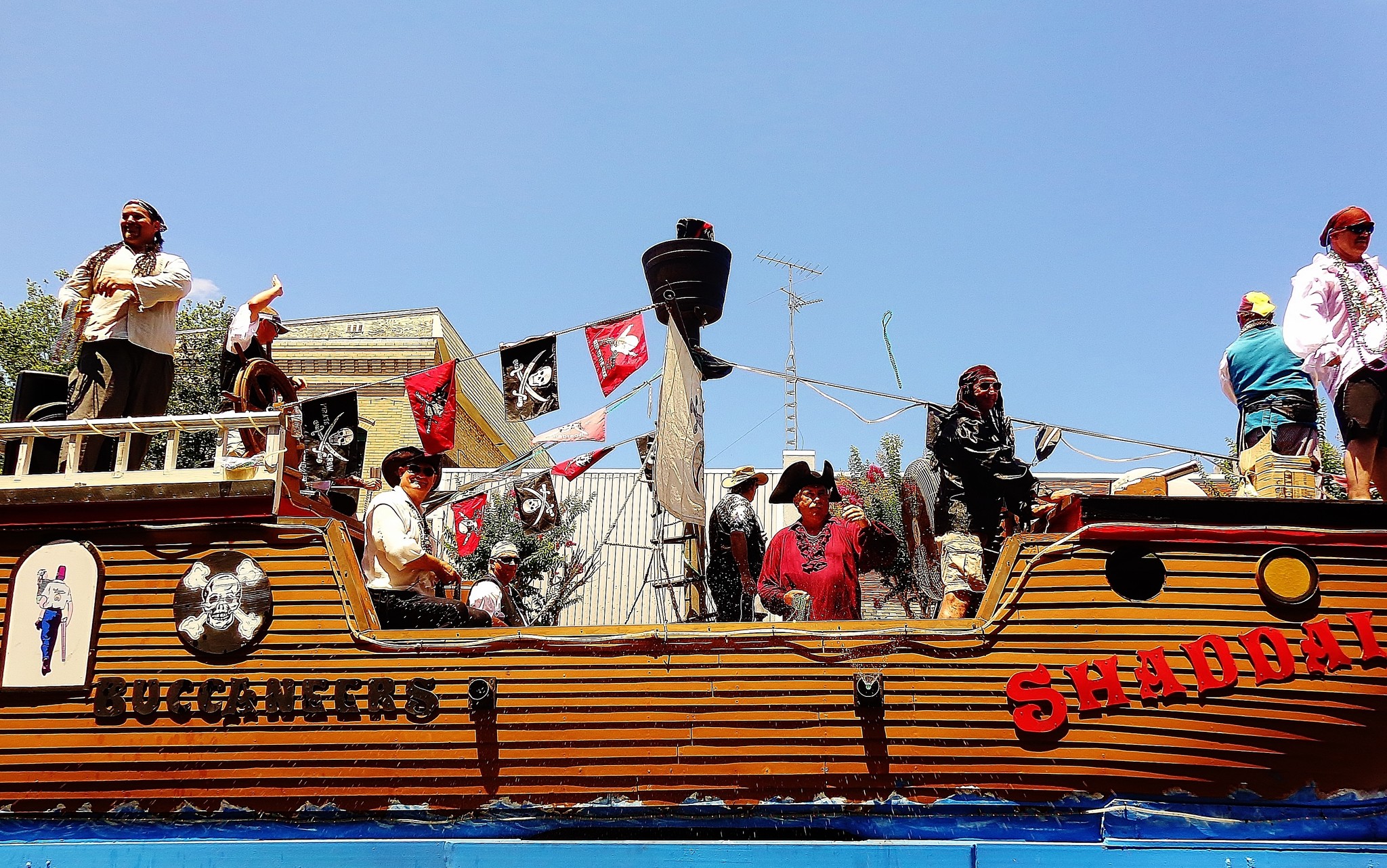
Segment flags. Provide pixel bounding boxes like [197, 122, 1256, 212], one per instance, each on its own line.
[585, 310, 648, 398]
[499, 332, 559, 422]
[403, 357, 456, 456]
[513, 469, 562, 537]
[300, 390, 359, 479]
[450, 490, 488, 557]
[529, 408, 655, 491]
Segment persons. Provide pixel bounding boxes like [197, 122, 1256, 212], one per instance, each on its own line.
[57, 199, 193, 473]
[362, 446, 492, 627]
[298, 451, 382, 518]
[467, 541, 528, 627]
[705, 465, 769, 621]
[217, 275, 308, 458]
[933, 364, 1089, 618]
[1283, 206, 1387, 500]
[758, 459, 899, 620]
[1220, 291, 1327, 500]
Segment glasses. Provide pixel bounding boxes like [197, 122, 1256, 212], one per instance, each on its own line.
[495, 556, 521, 565]
[977, 381, 1002, 390]
[1336, 221, 1375, 235]
[407, 463, 437, 477]
[269, 320, 280, 333]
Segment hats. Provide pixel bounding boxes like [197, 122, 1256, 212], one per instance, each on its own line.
[769, 460, 843, 504]
[258, 312, 291, 335]
[490, 541, 519, 557]
[381, 446, 445, 493]
[721, 465, 769, 488]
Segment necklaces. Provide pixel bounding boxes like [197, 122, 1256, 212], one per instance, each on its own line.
[1327, 250, 1387, 370]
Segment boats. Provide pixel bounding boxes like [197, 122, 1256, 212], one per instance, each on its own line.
[5, 217, 1387, 819]
